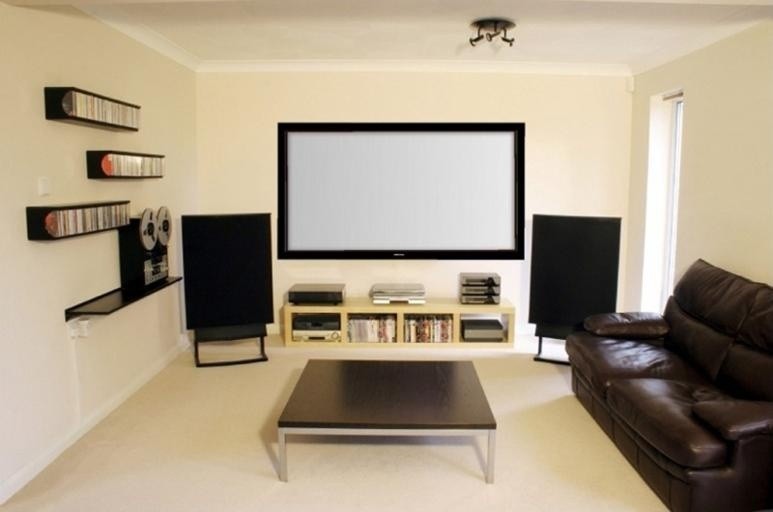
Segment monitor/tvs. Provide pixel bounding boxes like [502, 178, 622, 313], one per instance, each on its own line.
[277, 122, 525, 260]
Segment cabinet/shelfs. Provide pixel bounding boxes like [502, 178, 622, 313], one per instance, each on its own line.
[28, 85, 183, 321]
[283, 299, 513, 348]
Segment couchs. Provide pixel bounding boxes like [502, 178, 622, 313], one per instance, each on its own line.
[567, 260, 772, 512]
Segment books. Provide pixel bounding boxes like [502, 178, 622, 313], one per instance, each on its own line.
[102, 154, 165, 177]
[63, 90, 140, 129]
[348, 313, 455, 344]
[45, 204, 131, 238]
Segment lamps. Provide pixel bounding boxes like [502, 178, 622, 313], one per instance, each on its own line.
[470, 20, 516, 49]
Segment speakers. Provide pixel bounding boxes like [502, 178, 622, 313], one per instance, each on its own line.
[180, 213, 276, 340]
[528, 214, 622, 339]
